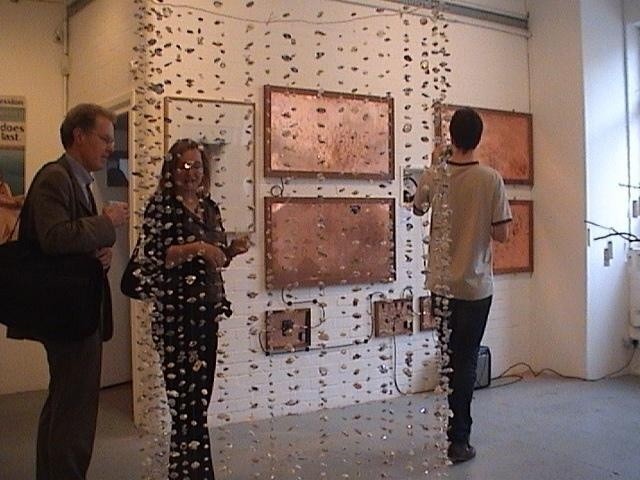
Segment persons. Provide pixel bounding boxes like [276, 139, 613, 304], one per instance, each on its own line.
[413, 108, 513, 465]
[142, 138, 252, 480]
[17, 103, 131, 480]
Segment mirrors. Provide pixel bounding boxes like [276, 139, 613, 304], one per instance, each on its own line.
[105, 113, 127, 188]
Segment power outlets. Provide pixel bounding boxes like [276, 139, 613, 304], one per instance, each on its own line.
[628, 337, 639, 348]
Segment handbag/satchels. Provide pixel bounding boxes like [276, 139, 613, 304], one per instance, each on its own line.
[119, 260, 152, 300]
[0, 240, 104, 340]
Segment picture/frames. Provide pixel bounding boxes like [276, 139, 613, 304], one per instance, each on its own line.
[163, 97, 256, 235]
[437, 104, 533, 187]
[491, 199, 534, 274]
[263, 195, 396, 290]
[263, 83, 395, 180]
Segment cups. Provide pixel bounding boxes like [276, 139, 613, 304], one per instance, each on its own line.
[234, 224, 250, 248]
[102, 198, 117, 211]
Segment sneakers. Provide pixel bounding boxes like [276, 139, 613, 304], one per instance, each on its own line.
[446, 443, 476, 464]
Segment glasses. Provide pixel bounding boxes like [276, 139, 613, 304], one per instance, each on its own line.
[84, 127, 115, 147]
